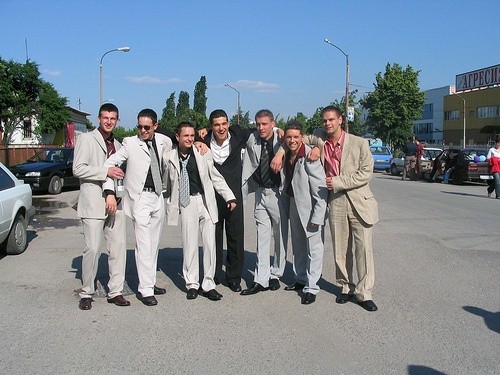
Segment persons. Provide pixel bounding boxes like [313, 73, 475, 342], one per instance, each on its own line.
[402, 136, 419, 181]
[274, 120, 334, 305]
[441, 154, 460, 184]
[155, 121, 238, 300]
[240, 109, 325, 297]
[412, 136, 425, 181]
[192, 110, 285, 292]
[270, 105, 381, 311]
[486, 138, 500, 198]
[102, 109, 174, 306]
[72, 103, 131, 310]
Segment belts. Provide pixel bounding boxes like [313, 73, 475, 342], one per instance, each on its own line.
[259, 184, 278, 188]
[143, 187, 156, 193]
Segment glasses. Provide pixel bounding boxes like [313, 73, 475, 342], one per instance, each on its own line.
[137, 124, 154, 130]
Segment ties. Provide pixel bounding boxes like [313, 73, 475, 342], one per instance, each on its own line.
[179, 154, 190, 208]
[147, 141, 162, 196]
[261, 142, 271, 187]
[107, 137, 115, 157]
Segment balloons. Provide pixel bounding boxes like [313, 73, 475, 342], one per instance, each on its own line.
[474, 156, 480, 162]
[480, 155, 486, 162]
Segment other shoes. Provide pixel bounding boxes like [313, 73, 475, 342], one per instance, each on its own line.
[487, 189, 491, 197]
[496, 197, 500, 199]
[438, 176, 444, 179]
[441, 180, 448, 183]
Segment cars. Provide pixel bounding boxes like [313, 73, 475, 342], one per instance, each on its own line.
[430, 147, 495, 185]
[0, 161, 40, 254]
[370, 145, 393, 173]
[390, 148, 446, 180]
[8, 147, 81, 194]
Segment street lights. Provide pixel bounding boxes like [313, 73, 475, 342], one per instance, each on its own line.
[225, 83, 240, 125]
[99, 46, 131, 106]
[323, 37, 349, 133]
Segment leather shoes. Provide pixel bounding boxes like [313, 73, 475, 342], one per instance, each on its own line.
[240, 282, 269, 295]
[136, 291, 157, 305]
[359, 300, 377, 311]
[199, 287, 223, 300]
[284, 282, 305, 290]
[107, 295, 130, 306]
[301, 292, 316, 305]
[336, 293, 351, 304]
[187, 288, 199, 299]
[269, 279, 280, 290]
[228, 283, 241, 292]
[154, 286, 166, 295]
[79, 298, 92, 310]
